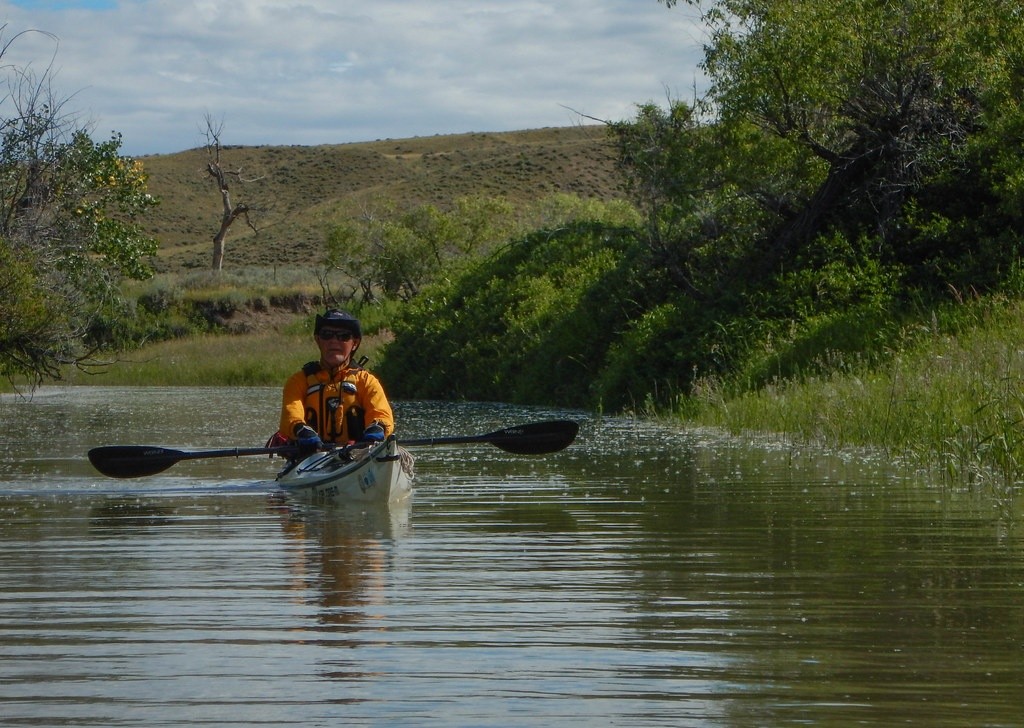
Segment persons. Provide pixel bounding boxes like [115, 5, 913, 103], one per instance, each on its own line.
[278, 308, 394, 453]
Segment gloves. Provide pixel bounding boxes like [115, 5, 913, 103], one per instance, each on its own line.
[362, 425, 384, 442]
[297, 429, 323, 450]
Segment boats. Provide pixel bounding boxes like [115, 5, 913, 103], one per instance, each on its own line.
[272, 433, 416, 504]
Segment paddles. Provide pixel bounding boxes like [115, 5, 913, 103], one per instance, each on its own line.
[86, 418, 582, 480]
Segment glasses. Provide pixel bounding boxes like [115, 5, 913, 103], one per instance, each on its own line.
[318, 328, 354, 342]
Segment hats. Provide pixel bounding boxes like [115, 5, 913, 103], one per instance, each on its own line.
[314, 309, 362, 357]
